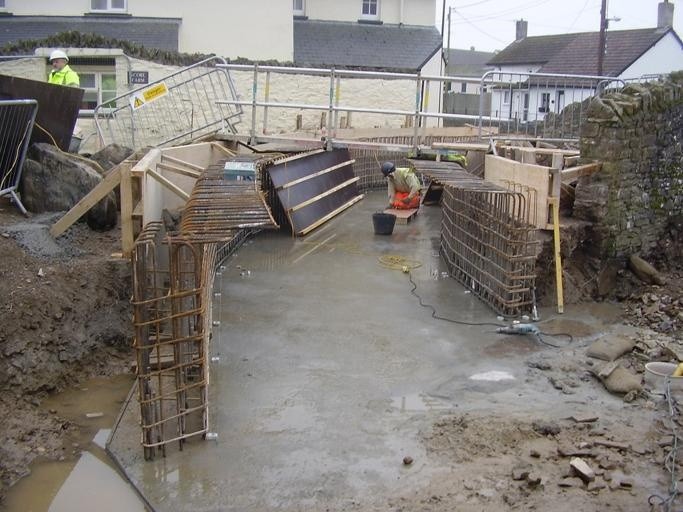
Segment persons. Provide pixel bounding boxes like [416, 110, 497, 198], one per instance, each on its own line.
[95, 71, 116, 109]
[380, 161, 422, 210]
[44, 48, 80, 136]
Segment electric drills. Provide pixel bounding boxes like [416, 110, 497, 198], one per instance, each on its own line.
[495, 323, 540, 335]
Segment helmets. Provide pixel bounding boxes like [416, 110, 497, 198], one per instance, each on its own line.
[49, 51, 69, 62]
[381, 162, 394, 177]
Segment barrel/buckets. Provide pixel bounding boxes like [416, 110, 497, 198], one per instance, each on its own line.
[645, 362, 683, 394]
[371, 208, 397, 235]
